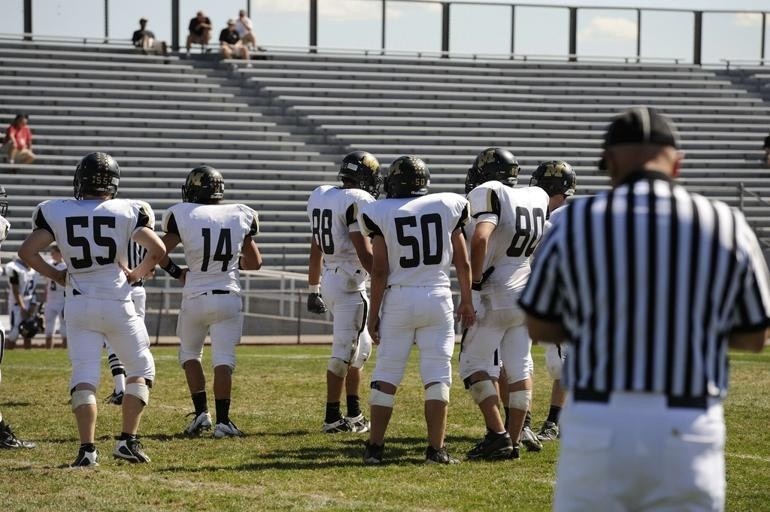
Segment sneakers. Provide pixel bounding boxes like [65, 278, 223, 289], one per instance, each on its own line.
[466, 427, 514, 458]
[520, 426, 543, 451]
[535, 420, 559, 440]
[184, 410, 212, 436]
[246, 63, 253, 68]
[69, 444, 101, 467]
[102, 388, 124, 407]
[214, 420, 245, 438]
[424, 446, 462, 465]
[323, 412, 368, 433]
[508, 442, 520, 461]
[345, 412, 371, 432]
[230, 63, 239, 70]
[0, 424, 37, 449]
[112, 440, 151, 464]
[362, 438, 386, 465]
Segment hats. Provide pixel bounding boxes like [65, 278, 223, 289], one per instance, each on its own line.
[597, 108, 675, 171]
[226, 18, 238, 25]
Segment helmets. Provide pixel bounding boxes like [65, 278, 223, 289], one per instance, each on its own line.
[529, 160, 577, 197]
[464, 148, 519, 194]
[182, 166, 225, 205]
[382, 156, 430, 199]
[17, 314, 45, 339]
[71, 152, 121, 200]
[337, 151, 384, 200]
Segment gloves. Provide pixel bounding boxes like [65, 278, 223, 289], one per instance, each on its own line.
[307, 293, 328, 315]
[471, 266, 495, 291]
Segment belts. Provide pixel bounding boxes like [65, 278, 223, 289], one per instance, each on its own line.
[201, 289, 230, 295]
[63, 288, 82, 297]
[571, 388, 708, 410]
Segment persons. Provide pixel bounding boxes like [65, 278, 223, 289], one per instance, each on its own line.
[361, 157, 478, 466]
[132, 18, 155, 54]
[159, 161, 261, 444]
[528, 160, 578, 437]
[186, 12, 212, 57]
[19, 150, 167, 466]
[306, 152, 376, 437]
[1, 114, 35, 165]
[219, 20, 252, 71]
[44, 243, 69, 347]
[237, 9, 266, 51]
[5, 261, 35, 350]
[463, 144, 543, 464]
